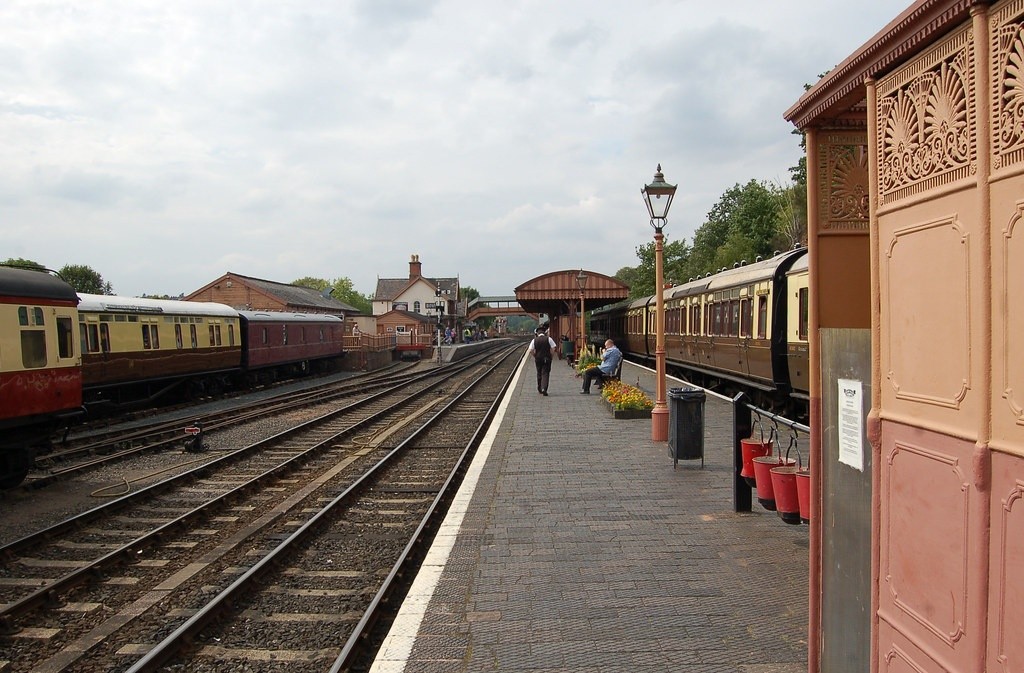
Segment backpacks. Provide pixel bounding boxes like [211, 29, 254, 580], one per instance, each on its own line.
[465, 331, 468, 335]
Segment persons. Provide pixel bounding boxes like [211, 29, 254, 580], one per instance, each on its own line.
[444, 325, 486, 343]
[529, 330, 557, 395]
[578, 339, 619, 393]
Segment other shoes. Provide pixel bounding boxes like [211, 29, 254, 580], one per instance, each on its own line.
[537, 385, 542, 394]
[598, 384, 606, 389]
[541, 389, 548, 396]
[580, 391, 590, 394]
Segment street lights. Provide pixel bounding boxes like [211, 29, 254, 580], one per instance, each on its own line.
[575, 267, 588, 353]
[640, 161, 678, 441]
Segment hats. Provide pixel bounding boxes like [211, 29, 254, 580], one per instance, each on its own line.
[535, 327, 546, 332]
[354, 324, 358, 327]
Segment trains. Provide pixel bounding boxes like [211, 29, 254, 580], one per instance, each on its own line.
[1, 264, 344, 491]
[585, 241, 809, 424]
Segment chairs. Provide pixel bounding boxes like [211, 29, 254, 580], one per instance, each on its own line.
[602, 356, 624, 388]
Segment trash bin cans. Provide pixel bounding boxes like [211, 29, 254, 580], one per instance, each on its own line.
[666, 385, 708, 470]
